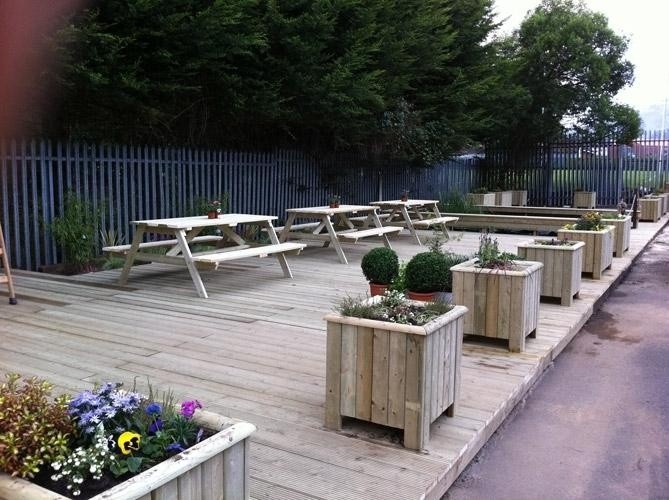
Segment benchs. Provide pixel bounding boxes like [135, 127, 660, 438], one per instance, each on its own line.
[191, 242, 307, 263]
[413, 217, 460, 226]
[347, 213, 391, 221]
[261, 222, 334, 232]
[336, 226, 404, 238]
[101, 235, 224, 254]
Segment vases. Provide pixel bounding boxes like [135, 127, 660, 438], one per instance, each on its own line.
[1, 390, 257, 500]
[574, 191, 596, 209]
[321, 294, 470, 450]
[636, 192, 669, 222]
[488, 190, 527, 206]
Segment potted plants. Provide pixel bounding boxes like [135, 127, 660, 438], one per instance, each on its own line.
[449, 234, 545, 354]
[405, 251, 448, 303]
[359, 247, 399, 297]
[466, 186, 496, 206]
[557, 209, 616, 280]
[518, 239, 585, 307]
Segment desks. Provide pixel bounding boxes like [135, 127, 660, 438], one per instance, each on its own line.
[278, 204, 404, 265]
[101, 213, 307, 299]
[369, 199, 460, 246]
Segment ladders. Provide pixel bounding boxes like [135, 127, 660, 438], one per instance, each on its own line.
[0, 224, 17, 305]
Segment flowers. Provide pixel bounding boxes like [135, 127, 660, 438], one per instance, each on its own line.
[0, 371, 204, 498]
[330, 288, 454, 326]
